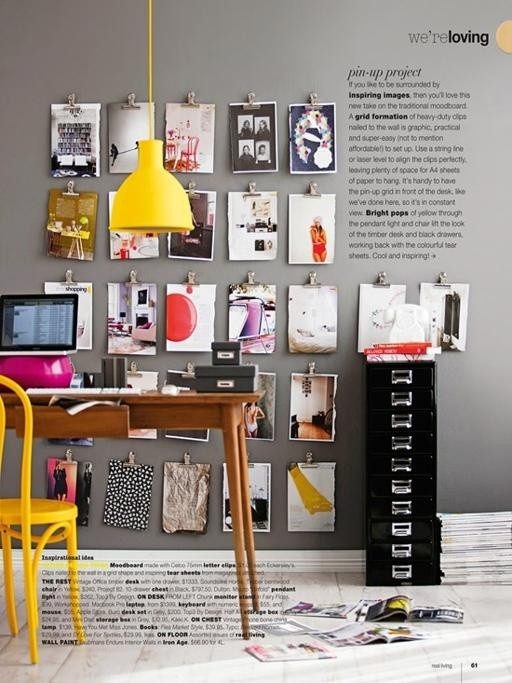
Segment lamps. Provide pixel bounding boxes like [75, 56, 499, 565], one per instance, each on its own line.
[108, 0, 195, 232]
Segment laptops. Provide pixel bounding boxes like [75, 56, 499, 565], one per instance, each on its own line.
[0, 294, 79, 356]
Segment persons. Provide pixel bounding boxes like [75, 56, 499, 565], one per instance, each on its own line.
[256, 120, 269, 136]
[241, 121, 253, 137]
[84, 463, 92, 505]
[310, 217, 327, 261]
[181, 203, 201, 243]
[256, 145, 271, 164]
[53, 461, 68, 501]
[244, 402, 265, 438]
[239, 145, 254, 164]
[111, 144, 118, 165]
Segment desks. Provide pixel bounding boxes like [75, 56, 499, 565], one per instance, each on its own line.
[0, 388, 266, 640]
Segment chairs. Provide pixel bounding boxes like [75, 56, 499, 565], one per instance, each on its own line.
[0, 375, 85, 666]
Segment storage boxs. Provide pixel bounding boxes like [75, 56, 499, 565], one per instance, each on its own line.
[195, 365, 257, 393]
[365, 362, 440, 586]
[211, 342, 240, 365]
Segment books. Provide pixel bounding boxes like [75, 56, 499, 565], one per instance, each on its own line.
[436, 510, 512, 585]
